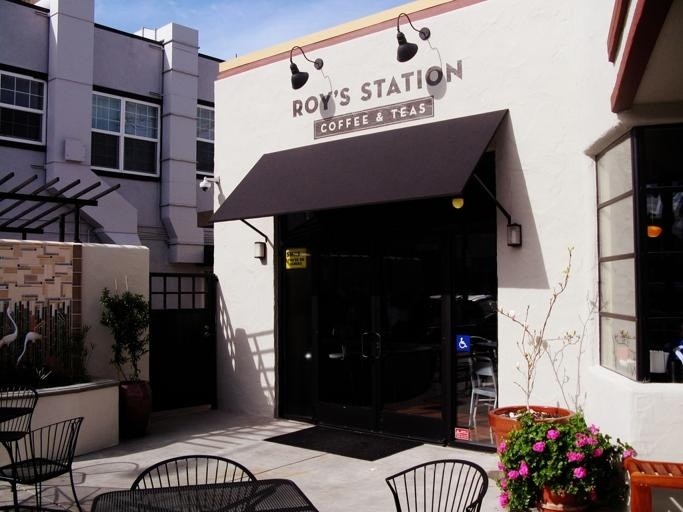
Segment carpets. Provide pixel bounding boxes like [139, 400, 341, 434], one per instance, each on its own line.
[264, 425, 427, 462]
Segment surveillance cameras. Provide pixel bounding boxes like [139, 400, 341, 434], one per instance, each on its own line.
[200, 177, 211, 192]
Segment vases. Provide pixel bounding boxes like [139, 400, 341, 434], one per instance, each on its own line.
[540, 474, 594, 511]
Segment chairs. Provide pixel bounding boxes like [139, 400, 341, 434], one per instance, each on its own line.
[129, 455, 257, 490]
[384, 457, 491, 512]
[0, 384, 40, 491]
[462, 336, 498, 430]
[0, 417, 86, 512]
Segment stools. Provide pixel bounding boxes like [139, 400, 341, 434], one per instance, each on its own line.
[625, 452, 683, 509]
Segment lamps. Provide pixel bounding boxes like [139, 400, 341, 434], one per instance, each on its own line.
[289, 46, 322, 90]
[396, 12, 432, 62]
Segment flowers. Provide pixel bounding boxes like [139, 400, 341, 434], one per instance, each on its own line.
[495, 413, 638, 512]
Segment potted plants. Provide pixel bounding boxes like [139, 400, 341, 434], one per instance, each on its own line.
[488, 247, 582, 460]
[98, 287, 155, 438]
